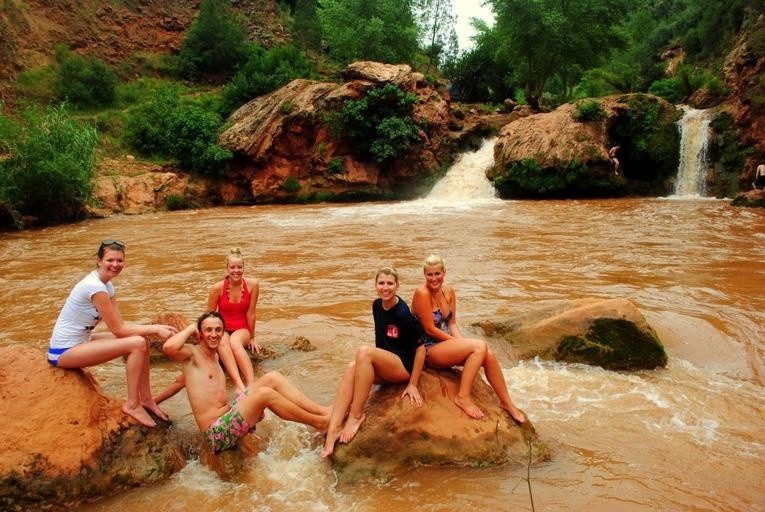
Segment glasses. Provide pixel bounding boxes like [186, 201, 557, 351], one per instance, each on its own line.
[102, 239, 125, 246]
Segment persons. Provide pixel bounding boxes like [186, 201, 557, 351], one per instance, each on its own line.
[154, 312, 333, 451]
[317, 266, 428, 458]
[206, 246, 263, 394]
[411, 255, 526, 423]
[46, 241, 179, 429]
